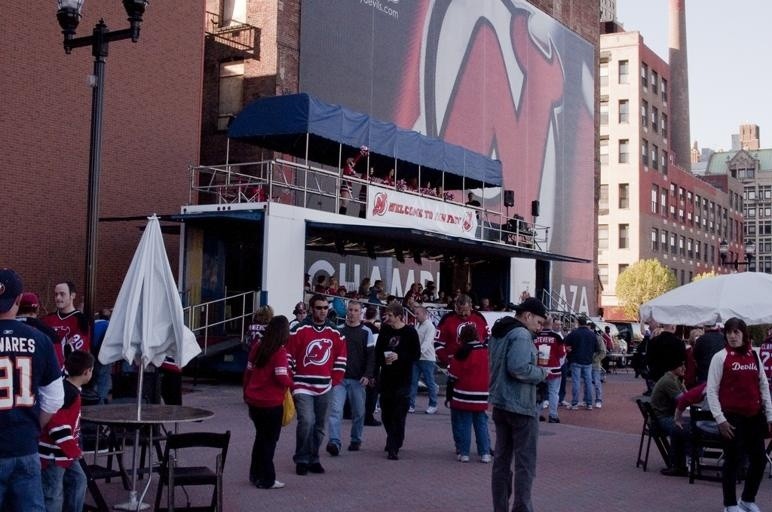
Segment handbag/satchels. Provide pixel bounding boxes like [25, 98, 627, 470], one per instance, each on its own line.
[759, 406, 772, 439]
[282, 386, 296, 425]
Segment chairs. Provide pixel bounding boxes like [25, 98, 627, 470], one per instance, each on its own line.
[689, 405, 744, 485]
[153, 430, 232, 512]
[636, 399, 671, 472]
[103, 371, 163, 490]
[78, 389, 101, 451]
[80, 457, 110, 512]
[765, 438, 772, 478]
[160, 373, 183, 406]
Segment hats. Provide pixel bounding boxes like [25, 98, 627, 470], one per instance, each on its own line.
[506, 298, 549, 319]
[703, 320, 718, 330]
[20, 292, 39, 308]
[0, 267, 23, 312]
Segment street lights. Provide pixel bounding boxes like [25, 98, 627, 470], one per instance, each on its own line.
[55, 1, 151, 453]
[717, 237, 757, 271]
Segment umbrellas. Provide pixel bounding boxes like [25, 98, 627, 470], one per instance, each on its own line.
[638, 271, 772, 328]
[98, 213, 203, 410]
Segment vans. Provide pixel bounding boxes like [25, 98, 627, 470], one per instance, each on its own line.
[611, 319, 650, 354]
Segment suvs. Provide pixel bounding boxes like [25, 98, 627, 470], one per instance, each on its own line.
[590, 320, 630, 368]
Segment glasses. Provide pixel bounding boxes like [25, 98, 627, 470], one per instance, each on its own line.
[315, 306, 329, 310]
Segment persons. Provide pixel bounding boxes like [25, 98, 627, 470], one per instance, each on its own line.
[434, 287, 613, 462]
[242, 275, 438, 489]
[617, 317, 772, 512]
[487, 297, 552, 511]
[217, 146, 482, 239]
[1, 269, 182, 511]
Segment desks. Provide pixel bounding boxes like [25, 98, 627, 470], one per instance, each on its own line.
[81, 404, 216, 512]
[609, 353, 634, 375]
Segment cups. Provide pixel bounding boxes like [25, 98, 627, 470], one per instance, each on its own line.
[384, 351, 393, 365]
[538, 344, 551, 366]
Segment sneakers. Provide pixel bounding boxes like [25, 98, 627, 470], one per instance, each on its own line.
[542, 400, 603, 410]
[408, 405, 416, 413]
[661, 468, 688, 476]
[327, 443, 338, 455]
[364, 418, 380, 426]
[296, 462, 308, 474]
[348, 441, 359, 451]
[549, 415, 560, 423]
[737, 496, 760, 512]
[480, 455, 491, 463]
[724, 506, 746, 512]
[249, 475, 285, 488]
[308, 462, 324, 473]
[425, 405, 438, 414]
[457, 454, 470, 463]
